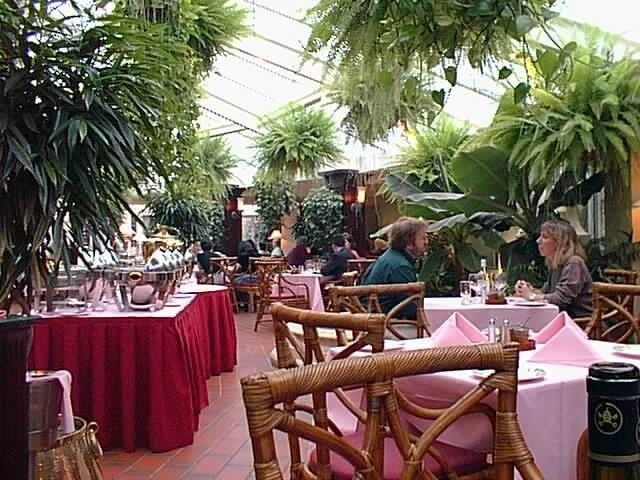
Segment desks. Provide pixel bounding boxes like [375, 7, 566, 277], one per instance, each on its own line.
[33, 295, 203, 453]
[178, 282, 238, 377]
[331, 330, 640, 479]
[421, 294, 560, 334]
[271, 265, 324, 312]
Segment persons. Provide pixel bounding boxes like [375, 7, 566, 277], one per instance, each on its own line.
[356, 216, 429, 320]
[196, 237, 226, 274]
[231, 237, 259, 314]
[343, 231, 360, 261]
[183, 239, 194, 262]
[270, 238, 283, 257]
[194, 240, 205, 261]
[318, 232, 360, 294]
[285, 235, 308, 270]
[513, 219, 595, 317]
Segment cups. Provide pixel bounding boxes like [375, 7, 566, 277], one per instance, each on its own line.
[458, 281, 473, 306]
[511, 325, 529, 342]
[467, 274, 489, 304]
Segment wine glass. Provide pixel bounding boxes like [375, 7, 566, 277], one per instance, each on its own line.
[492, 271, 509, 299]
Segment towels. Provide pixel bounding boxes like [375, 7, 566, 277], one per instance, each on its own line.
[432, 312, 487, 345]
[527, 310, 599, 361]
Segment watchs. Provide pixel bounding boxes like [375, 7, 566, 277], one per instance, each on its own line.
[528, 293, 540, 301]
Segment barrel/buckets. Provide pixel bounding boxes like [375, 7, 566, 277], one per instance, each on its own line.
[28, 370, 64, 452]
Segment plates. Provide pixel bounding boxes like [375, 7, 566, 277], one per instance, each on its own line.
[617, 347, 640, 358]
[517, 301, 546, 307]
[473, 365, 546, 386]
[352, 340, 405, 350]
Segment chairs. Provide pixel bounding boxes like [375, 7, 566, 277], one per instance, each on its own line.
[584, 283, 640, 344]
[329, 280, 434, 347]
[241, 344, 544, 480]
[254, 260, 310, 331]
[574, 266, 638, 341]
[208, 256, 241, 315]
[322, 271, 361, 341]
[271, 303, 386, 480]
